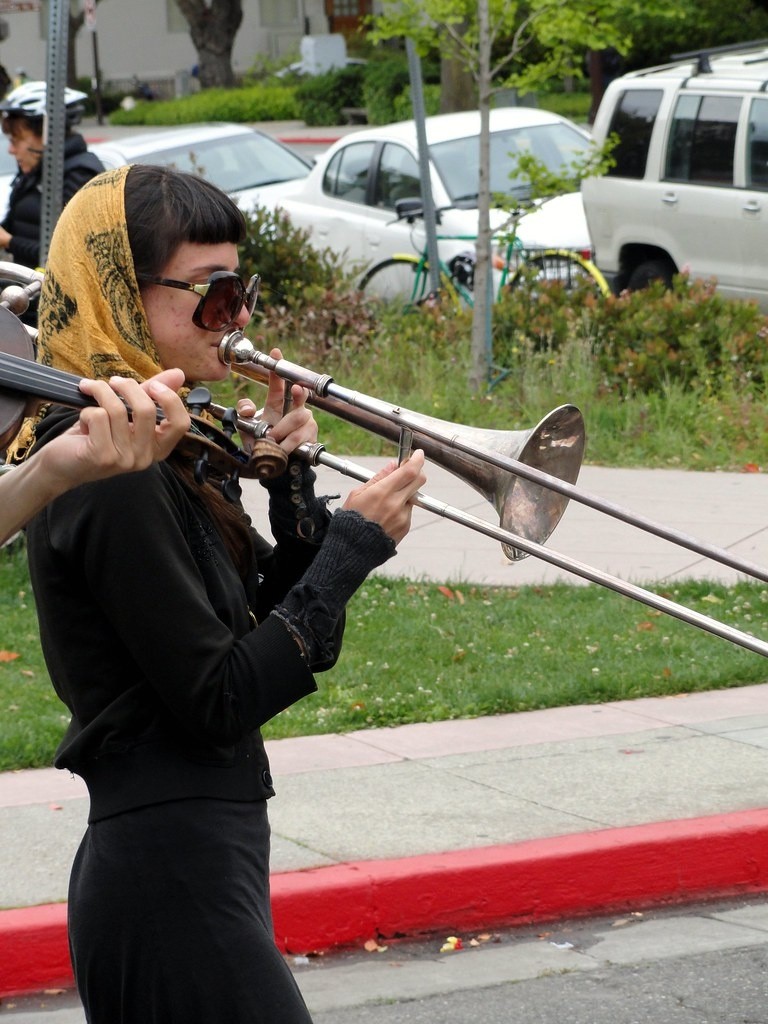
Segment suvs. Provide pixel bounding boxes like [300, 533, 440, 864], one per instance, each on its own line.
[578, 37, 767, 316]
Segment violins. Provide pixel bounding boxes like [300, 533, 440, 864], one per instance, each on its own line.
[0, 302, 290, 482]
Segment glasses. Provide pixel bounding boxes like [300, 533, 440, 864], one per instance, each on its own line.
[136, 272, 261, 334]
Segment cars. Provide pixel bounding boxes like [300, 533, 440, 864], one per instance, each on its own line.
[273, 104, 591, 310]
[0, 119, 324, 245]
[276, 56, 367, 80]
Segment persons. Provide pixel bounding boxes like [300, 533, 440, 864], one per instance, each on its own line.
[0, 81, 105, 362]
[0, 163, 427, 1024]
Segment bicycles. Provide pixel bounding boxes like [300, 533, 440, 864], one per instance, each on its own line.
[354, 205, 618, 344]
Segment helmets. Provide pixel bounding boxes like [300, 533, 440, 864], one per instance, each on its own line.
[0, 82, 88, 129]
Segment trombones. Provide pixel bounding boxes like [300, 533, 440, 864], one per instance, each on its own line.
[209, 330, 768, 660]
